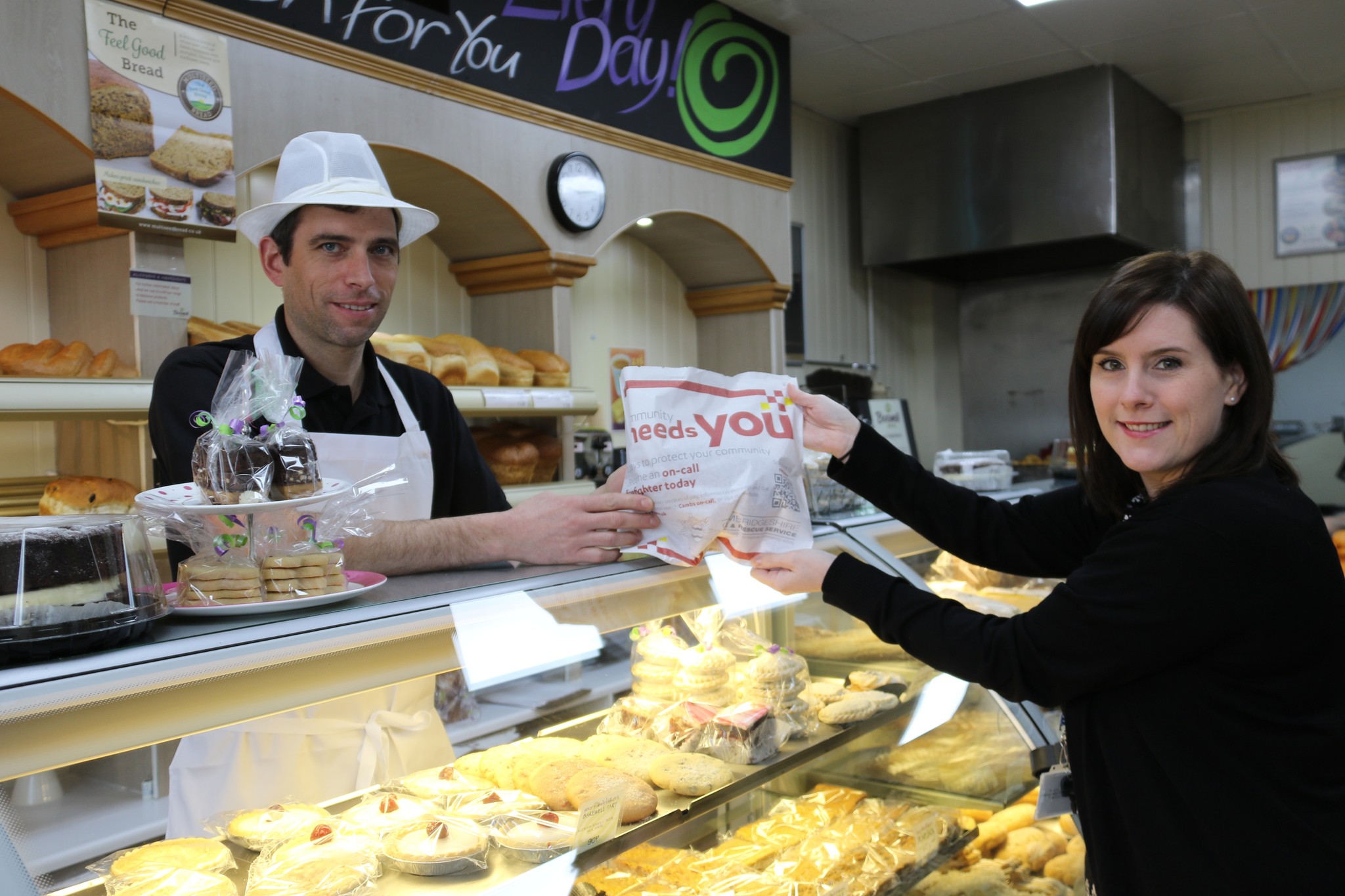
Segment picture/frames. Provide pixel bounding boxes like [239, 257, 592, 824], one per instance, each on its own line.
[1267, 148, 1345, 259]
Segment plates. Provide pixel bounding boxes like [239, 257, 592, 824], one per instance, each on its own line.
[135, 478, 352, 507]
[133, 571, 388, 614]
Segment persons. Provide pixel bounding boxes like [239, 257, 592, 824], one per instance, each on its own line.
[143, 127, 664, 843]
[736, 247, 1345, 896]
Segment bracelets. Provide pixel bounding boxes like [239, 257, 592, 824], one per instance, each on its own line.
[835, 448, 852, 462]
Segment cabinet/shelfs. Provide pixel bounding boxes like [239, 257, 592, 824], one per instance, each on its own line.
[1, 460, 1134, 895]
[853, 63, 1187, 287]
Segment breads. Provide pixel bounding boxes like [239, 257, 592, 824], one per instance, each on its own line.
[466, 416, 560, 484]
[576, 781, 1086, 896]
[453, 735, 738, 823]
[187, 315, 572, 387]
[0, 338, 138, 380]
[866, 703, 1036, 800]
[37, 476, 142, 517]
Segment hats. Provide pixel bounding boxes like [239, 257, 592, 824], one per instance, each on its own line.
[235, 132, 440, 250]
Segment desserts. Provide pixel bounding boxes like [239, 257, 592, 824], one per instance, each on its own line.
[101, 765, 582, 896]
[602, 625, 909, 767]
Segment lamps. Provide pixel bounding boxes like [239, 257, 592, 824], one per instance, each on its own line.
[547, 150, 607, 233]
